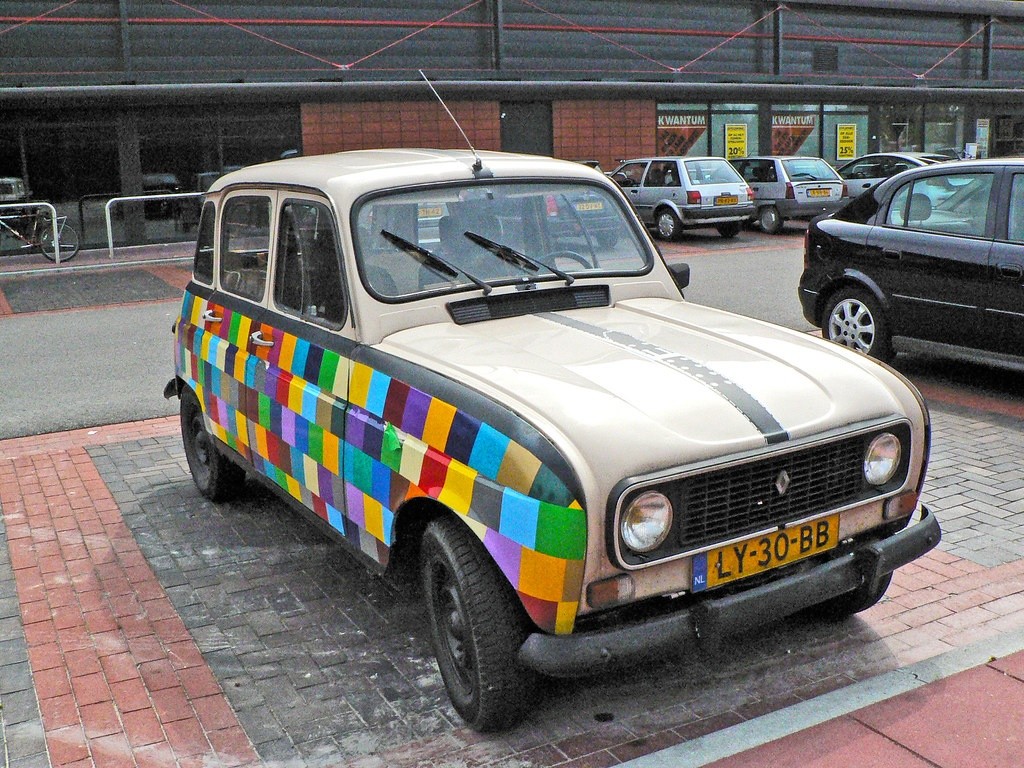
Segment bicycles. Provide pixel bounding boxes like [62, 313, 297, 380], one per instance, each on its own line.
[0, 200, 80, 262]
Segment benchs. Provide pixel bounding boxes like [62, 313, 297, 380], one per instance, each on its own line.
[901, 192, 986, 236]
[667, 169, 729, 186]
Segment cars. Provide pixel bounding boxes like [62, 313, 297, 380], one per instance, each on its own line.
[164, 148, 940, 734]
[606, 156, 758, 241]
[268, 191, 453, 248]
[1, 179, 36, 226]
[797, 157, 1024, 363]
[455, 161, 625, 249]
[174, 171, 237, 233]
[697, 156, 848, 235]
[114, 175, 180, 218]
[832, 151, 976, 212]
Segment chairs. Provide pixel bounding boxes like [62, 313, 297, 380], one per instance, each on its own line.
[648, 169, 665, 185]
[748, 166, 767, 182]
[311, 221, 399, 321]
[419, 214, 511, 287]
